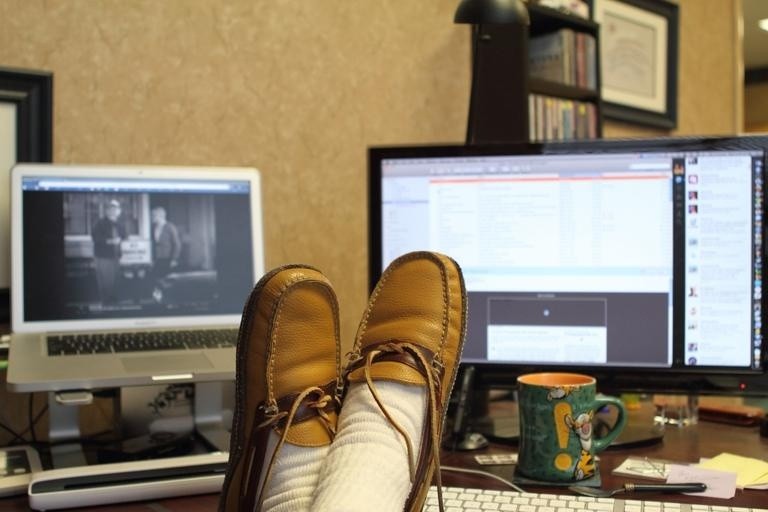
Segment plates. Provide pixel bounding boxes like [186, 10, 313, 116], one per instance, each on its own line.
[512, 466, 602, 488]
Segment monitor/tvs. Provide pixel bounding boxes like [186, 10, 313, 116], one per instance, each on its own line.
[368, 131, 768, 451]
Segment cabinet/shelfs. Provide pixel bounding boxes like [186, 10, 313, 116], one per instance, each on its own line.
[453, 1, 601, 147]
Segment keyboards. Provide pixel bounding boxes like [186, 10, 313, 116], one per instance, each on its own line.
[421, 485, 768, 512]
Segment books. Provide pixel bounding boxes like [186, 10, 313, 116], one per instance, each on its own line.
[526, 27, 599, 143]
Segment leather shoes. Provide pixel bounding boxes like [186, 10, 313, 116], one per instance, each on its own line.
[341, 249, 469, 512]
[216, 260, 346, 512]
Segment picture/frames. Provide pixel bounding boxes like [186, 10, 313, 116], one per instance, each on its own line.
[591, 1, 679, 130]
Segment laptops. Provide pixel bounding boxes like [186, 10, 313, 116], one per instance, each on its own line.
[5, 164, 266, 394]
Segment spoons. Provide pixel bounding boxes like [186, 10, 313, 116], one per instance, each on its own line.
[568, 483, 708, 498]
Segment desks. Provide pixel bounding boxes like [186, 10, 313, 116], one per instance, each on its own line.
[2, 389, 767, 510]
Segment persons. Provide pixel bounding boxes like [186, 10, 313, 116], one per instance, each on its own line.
[90, 200, 182, 303]
[216, 251, 468, 512]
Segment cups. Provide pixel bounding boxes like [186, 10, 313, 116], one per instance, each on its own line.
[515, 372, 626, 481]
[653, 393, 700, 427]
